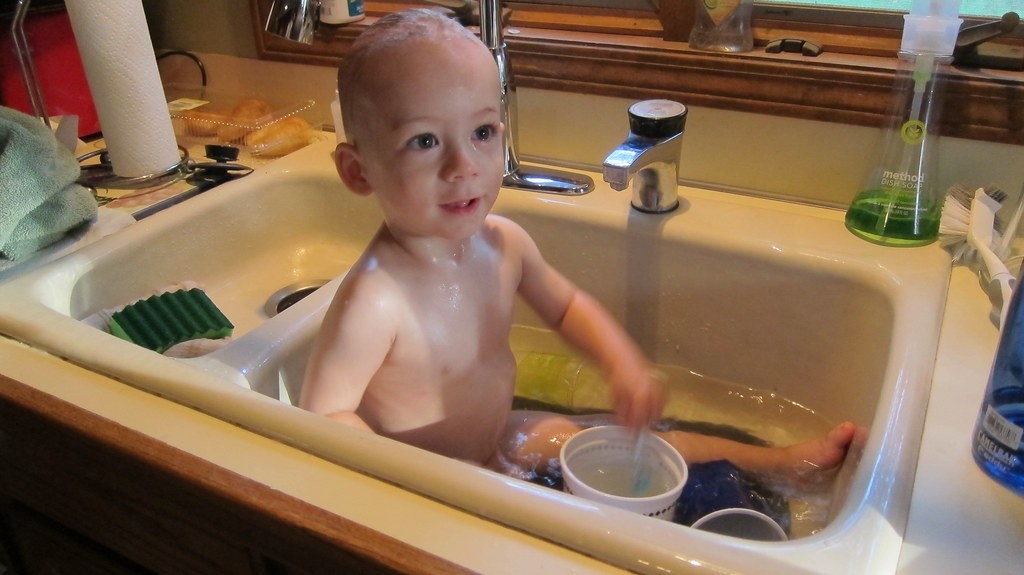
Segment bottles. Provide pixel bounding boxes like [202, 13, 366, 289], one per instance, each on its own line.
[688, 1, 753, 53]
[970, 258, 1024, 498]
[320, 0, 366, 24]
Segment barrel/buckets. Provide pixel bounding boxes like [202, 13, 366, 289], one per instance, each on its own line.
[690, 508, 788, 542]
[558, 425, 687, 523]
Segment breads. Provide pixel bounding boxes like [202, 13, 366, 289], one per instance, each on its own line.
[176, 98, 313, 158]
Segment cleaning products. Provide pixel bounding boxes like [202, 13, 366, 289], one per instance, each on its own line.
[843, 0, 965, 248]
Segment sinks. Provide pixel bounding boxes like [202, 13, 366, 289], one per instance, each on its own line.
[231, 189, 953, 574]
[30, 169, 397, 361]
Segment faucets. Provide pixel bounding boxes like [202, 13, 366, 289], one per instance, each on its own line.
[602, 98, 690, 213]
[479, 0, 596, 198]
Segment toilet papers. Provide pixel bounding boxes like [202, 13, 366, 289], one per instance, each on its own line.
[64, 0, 184, 178]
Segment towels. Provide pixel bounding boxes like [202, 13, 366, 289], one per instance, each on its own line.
[0, 104, 138, 288]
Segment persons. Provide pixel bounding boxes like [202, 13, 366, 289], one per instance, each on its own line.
[299, 9, 855, 465]
